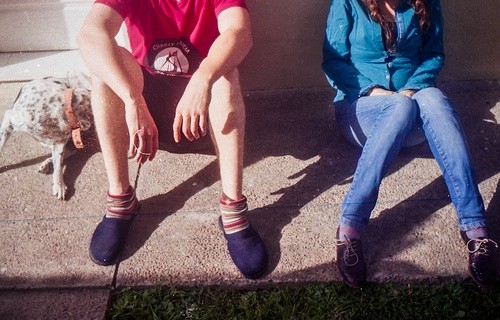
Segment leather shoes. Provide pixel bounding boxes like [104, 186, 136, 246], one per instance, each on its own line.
[333, 226, 368, 291]
[460, 227, 499, 287]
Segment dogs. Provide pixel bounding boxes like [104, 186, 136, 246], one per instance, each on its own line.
[0, 70, 95, 201]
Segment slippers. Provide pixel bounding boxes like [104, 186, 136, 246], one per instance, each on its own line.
[88, 204, 139, 267]
[217, 214, 268, 280]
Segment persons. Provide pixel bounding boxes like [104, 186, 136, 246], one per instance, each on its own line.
[321, 0, 499, 291]
[76, 0, 269, 280]
